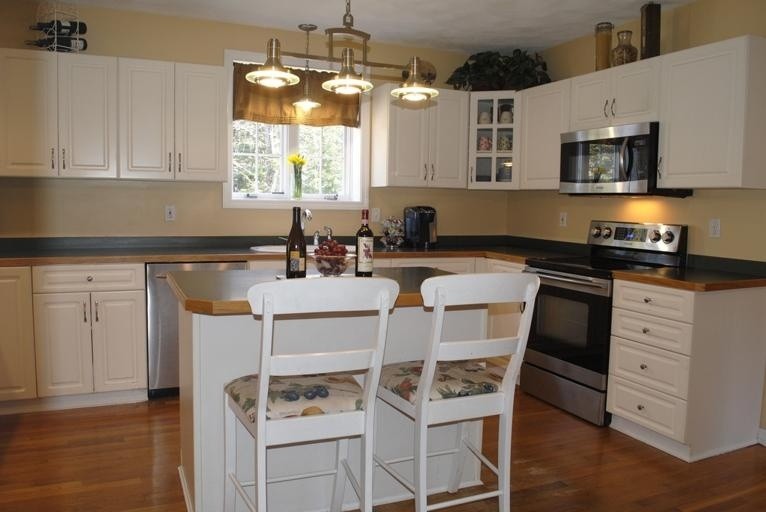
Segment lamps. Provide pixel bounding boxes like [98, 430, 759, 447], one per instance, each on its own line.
[246, 39, 299, 88]
[321, 0, 373, 95]
[389, 57, 438, 101]
[294, 24, 318, 110]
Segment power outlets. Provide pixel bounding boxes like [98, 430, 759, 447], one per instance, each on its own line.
[708, 219, 720, 237]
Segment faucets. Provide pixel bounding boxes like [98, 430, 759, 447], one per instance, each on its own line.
[280, 208, 313, 244]
[323, 226, 332, 240]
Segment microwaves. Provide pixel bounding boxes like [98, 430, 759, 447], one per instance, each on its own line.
[559, 121, 693, 198]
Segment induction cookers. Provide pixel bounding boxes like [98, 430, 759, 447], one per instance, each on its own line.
[525, 220, 688, 280]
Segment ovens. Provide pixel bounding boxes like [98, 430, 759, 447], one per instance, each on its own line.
[520, 267, 614, 427]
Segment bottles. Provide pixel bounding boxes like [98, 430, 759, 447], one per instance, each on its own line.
[594, 22, 614, 70]
[286, 207, 306, 279]
[29, 20, 86, 36]
[24, 37, 87, 51]
[355, 209, 374, 277]
[611, 31, 637, 67]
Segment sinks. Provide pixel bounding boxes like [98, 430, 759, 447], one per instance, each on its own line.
[249, 243, 357, 255]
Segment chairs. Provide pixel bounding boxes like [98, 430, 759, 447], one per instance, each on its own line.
[365, 273, 540, 512]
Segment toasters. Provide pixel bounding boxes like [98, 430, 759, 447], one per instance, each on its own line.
[403, 205, 438, 249]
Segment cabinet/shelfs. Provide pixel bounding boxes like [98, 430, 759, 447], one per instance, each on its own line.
[118, 59, 232, 180]
[372, 85, 468, 189]
[0, 266, 37, 400]
[521, 79, 576, 188]
[657, 34, 765, 187]
[223, 278, 400, 512]
[31, 263, 146, 397]
[0, 49, 117, 178]
[471, 91, 518, 189]
[572, 57, 660, 120]
[605, 280, 764, 461]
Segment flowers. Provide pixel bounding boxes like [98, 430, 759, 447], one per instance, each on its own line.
[288, 154, 305, 197]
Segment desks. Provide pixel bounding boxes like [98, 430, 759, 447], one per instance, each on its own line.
[167, 269, 486, 511]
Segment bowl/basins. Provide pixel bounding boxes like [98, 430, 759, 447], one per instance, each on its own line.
[306, 253, 358, 276]
